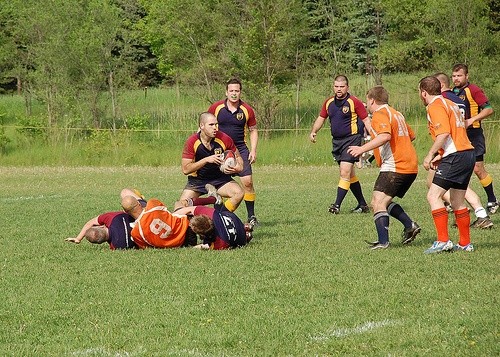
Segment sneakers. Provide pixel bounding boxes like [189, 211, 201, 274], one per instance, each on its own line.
[248, 216, 258, 226]
[485, 199, 500, 215]
[205, 184, 222, 205]
[244, 223, 253, 231]
[401, 222, 422, 244]
[451, 207, 470, 228]
[365, 239, 391, 251]
[469, 215, 493, 229]
[246, 231, 253, 243]
[454, 242, 474, 252]
[365, 159, 372, 165]
[350, 206, 370, 214]
[443, 202, 453, 212]
[328, 204, 340, 214]
[423, 240, 454, 254]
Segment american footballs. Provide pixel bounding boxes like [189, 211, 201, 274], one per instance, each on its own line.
[219, 150, 236, 172]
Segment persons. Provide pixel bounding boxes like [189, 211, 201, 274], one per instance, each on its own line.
[427, 73, 493, 228]
[178, 112, 244, 246]
[194, 79, 259, 228]
[65, 183, 258, 250]
[347, 85, 422, 250]
[355, 101, 375, 169]
[310, 75, 374, 214]
[450, 64, 500, 216]
[417, 77, 476, 255]
